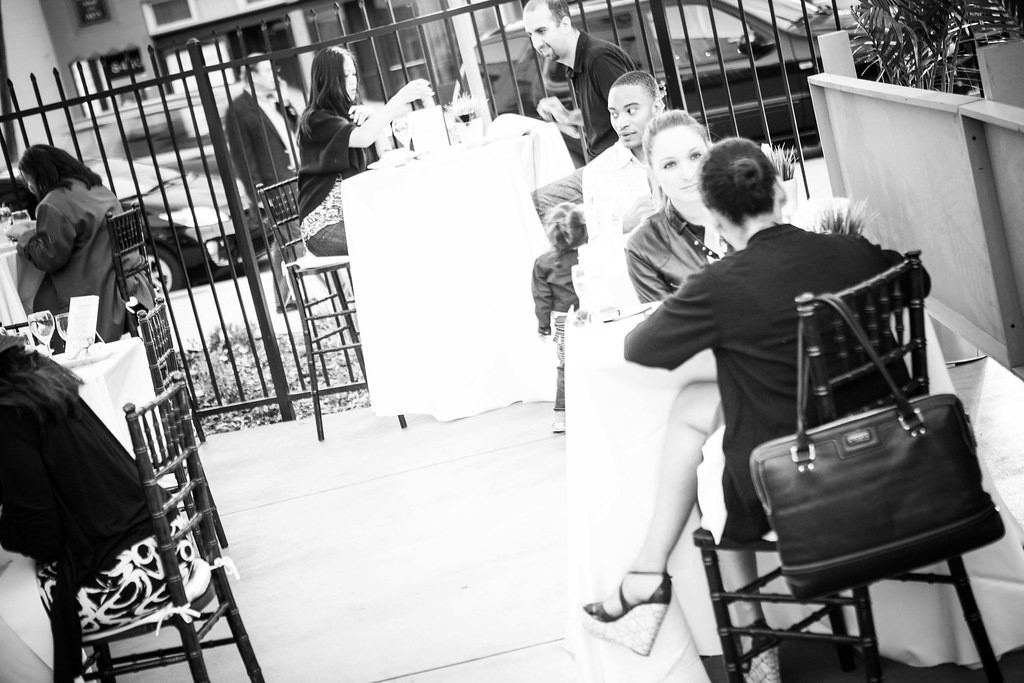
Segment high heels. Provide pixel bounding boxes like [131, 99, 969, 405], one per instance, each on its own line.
[581, 570, 673, 657]
[743, 619, 780, 683]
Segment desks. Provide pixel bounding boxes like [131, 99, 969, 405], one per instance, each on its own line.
[557, 294, 933, 683]
[0, 213, 39, 337]
[338, 121, 574, 418]
[51, 336, 166, 459]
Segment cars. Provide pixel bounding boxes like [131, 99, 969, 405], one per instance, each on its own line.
[1, 155, 276, 296]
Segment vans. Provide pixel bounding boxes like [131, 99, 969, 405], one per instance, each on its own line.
[39, 83, 311, 196]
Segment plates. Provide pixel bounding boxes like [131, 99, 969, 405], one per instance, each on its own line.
[367, 151, 421, 168]
[51, 347, 112, 367]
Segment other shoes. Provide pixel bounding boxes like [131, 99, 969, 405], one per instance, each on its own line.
[277, 296, 310, 312]
[551, 412, 565, 432]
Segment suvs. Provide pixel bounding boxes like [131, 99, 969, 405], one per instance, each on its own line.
[447, 0, 1005, 164]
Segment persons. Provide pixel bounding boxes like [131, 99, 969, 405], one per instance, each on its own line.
[626, 111, 744, 315]
[577, 136, 933, 683]
[5, 144, 133, 355]
[520, 0, 639, 166]
[1, 328, 196, 683]
[226, 52, 318, 314]
[296, 45, 434, 258]
[579, 70, 667, 246]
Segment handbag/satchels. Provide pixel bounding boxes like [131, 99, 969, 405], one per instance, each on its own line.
[749, 294, 1006, 605]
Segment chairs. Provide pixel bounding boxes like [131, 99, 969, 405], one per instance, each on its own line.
[254, 175, 408, 443]
[66, 370, 268, 683]
[687, 248, 1003, 683]
[134, 297, 230, 551]
[107, 204, 210, 449]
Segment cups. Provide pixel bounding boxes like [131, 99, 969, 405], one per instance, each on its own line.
[55, 312, 70, 341]
[0, 207, 31, 226]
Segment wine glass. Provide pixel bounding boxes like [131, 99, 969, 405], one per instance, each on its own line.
[28, 310, 55, 362]
[391, 117, 413, 151]
